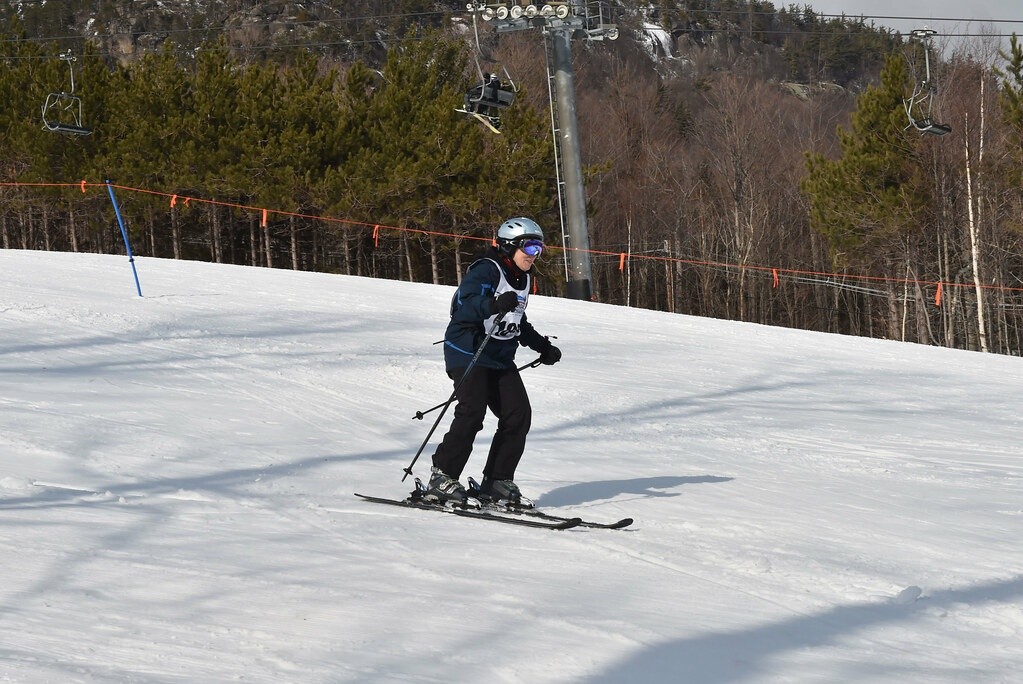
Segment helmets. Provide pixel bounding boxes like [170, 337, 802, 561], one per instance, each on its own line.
[496, 217, 544, 256]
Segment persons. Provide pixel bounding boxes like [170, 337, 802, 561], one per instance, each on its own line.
[429, 216, 563, 509]
[469, 72, 501, 118]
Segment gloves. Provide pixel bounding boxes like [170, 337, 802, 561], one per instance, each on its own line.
[540, 345, 561, 365]
[496, 291, 519, 312]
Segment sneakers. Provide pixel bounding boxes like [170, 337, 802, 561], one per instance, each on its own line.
[481, 475, 522, 503]
[428, 467, 468, 502]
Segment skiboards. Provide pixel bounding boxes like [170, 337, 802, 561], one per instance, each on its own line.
[351, 474, 636, 534]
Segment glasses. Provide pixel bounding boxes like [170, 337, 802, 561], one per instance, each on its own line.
[497, 238, 543, 256]
